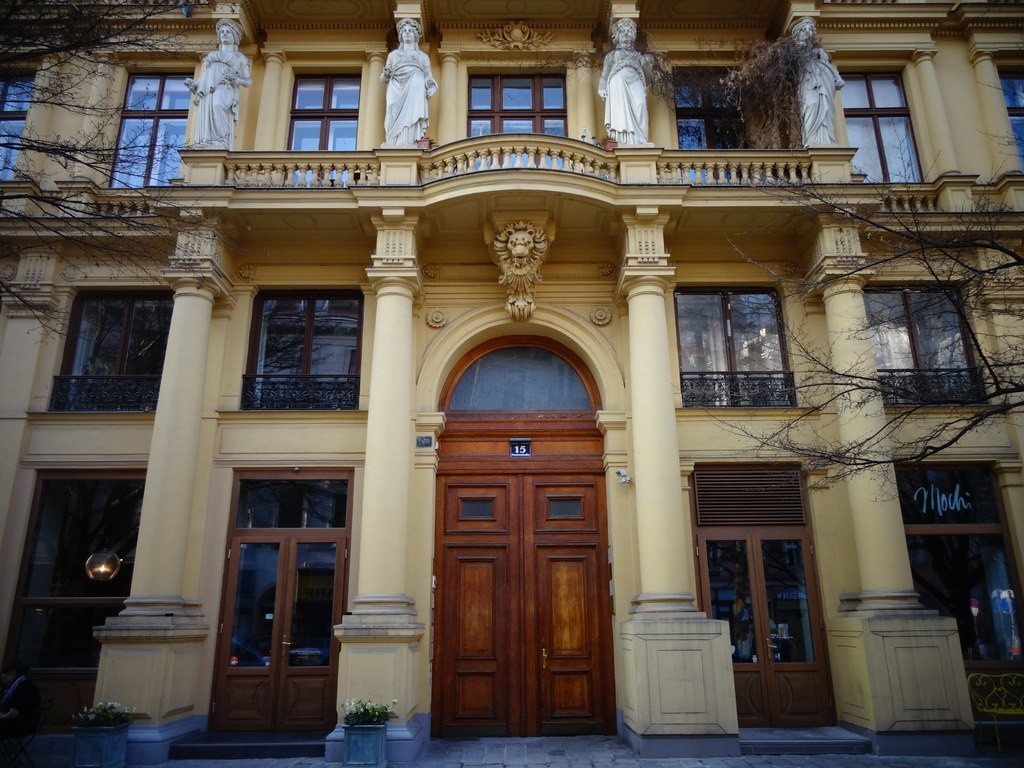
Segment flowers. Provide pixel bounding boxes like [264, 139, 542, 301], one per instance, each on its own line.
[69, 700, 138, 719]
[340, 697, 398, 723]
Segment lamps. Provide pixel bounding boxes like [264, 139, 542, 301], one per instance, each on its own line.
[85, 546, 122, 582]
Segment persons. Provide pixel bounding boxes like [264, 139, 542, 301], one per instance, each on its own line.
[379, 18, 438, 146]
[597, 18, 655, 145]
[792, 19, 846, 146]
[183, 19, 251, 150]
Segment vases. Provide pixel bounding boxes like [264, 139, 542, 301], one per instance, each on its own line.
[342, 723, 388, 768]
[69, 720, 133, 768]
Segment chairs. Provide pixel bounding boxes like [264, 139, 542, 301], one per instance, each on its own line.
[0, 685, 56, 768]
[966, 672, 1024, 753]
